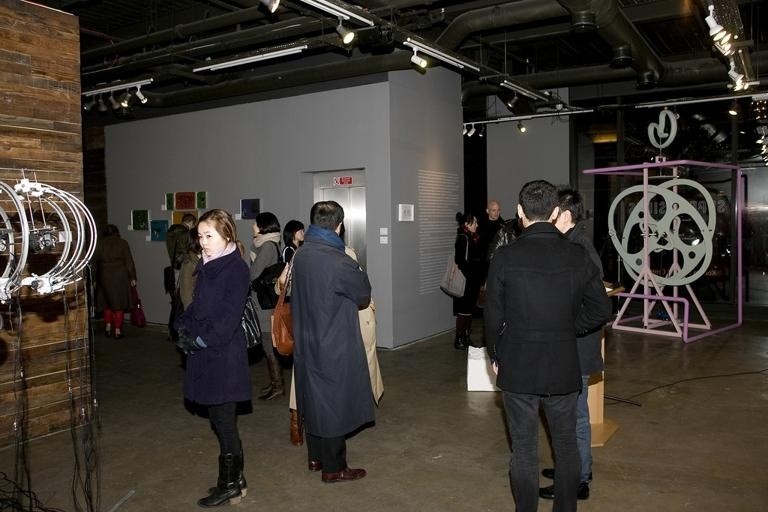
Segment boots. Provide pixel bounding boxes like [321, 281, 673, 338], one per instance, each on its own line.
[290, 409, 303, 447]
[209, 449, 248, 499]
[454, 315, 467, 352]
[258, 351, 285, 401]
[196, 452, 243, 510]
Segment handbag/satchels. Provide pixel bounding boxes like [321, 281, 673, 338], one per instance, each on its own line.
[252, 257, 287, 311]
[129, 292, 147, 328]
[271, 257, 293, 358]
[438, 248, 471, 299]
[237, 289, 263, 349]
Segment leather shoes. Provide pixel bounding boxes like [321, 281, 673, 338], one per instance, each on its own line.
[307, 458, 321, 471]
[115, 334, 124, 339]
[321, 467, 368, 484]
[537, 483, 590, 502]
[541, 467, 593, 481]
[102, 330, 111, 338]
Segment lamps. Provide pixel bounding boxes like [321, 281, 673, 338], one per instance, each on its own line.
[83, 95, 97, 111]
[108, 90, 121, 111]
[743, 83, 749, 90]
[728, 58, 745, 82]
[463, 124, 467, 135]
[714, 44, 731, 56]
[135, 82, 147, 104]
[120, 88, 132, 109]
[335, 15, 355, 45]
[98, 93, 107, 112]
[263, 0, 281, 14]
[507, 91, 518, 107]
[735, 79, 742, 85]
[719, 33, 732, 45]
[467, 123, 476, 137]
[728, 102, 741, 119]
[479, 126, 485, 137]
[705, 5, 723, 37]
[715, 40, 732, 50]
[410, 46, 428, 69]
[712, 29, 727, 42]
[517, 119, 526, 133]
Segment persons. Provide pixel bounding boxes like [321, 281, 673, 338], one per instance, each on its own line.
[482, 180, 613, 511]
[173, 209, 254, 507]
[537, 184, 606, 501]
[291, 200, 376, 483]
[477, 200, 505, 255]
[453, 212, 483, 350]
[166, 211, 384, 448]
[94, 224, 137, 340]
[488, 211, 525, 266]
